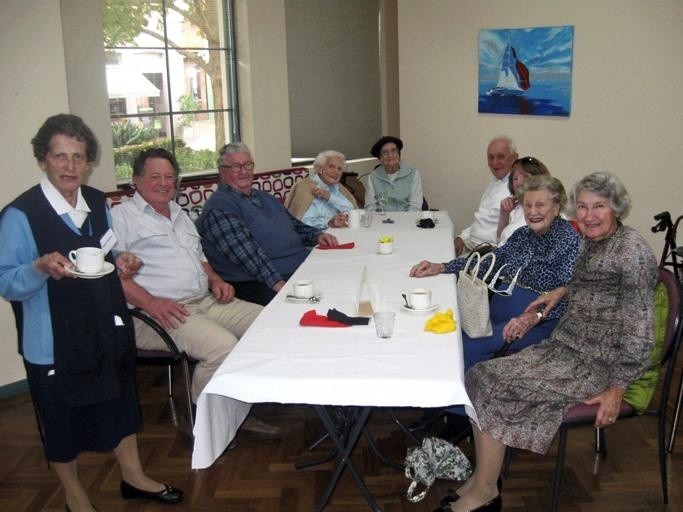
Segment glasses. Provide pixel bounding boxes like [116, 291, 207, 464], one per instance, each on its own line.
[488, 263, 522, 297]
[512, 157, 539, 168]
[220, 161, 254, 173]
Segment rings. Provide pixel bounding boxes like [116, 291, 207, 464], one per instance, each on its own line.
[609, 417, 613, 423]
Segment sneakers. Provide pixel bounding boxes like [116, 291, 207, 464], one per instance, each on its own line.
[64, 500, 101, 512]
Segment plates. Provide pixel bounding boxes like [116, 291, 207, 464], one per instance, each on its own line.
[63, 260, 115, 279]
[285, 290, 322, 303]
[400, 302, 439, 312]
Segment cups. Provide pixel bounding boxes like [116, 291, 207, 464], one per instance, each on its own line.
[411, 208, 441, 225]
[376, 241, 395, 255]
[291, 279, 316, 297]
[68, 247, 104, 275]
[409, 289, 432, 308]
[343, 208, 377, 228]
[371, 311, 396, 339]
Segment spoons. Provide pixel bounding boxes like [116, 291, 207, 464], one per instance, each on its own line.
[401, 293, 414, 309]
[286, 295, 321, 304]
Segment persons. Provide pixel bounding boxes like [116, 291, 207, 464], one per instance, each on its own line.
[286, 150, 354, 230]
[455, 136, 519, 258]
[433, 172, 656, 512]
[365, 136, 423, 211]
[0, 113, 184, 511]
[409, 173, 578, 369]
[108, 146, 279, 451]
[195, 142, 336, 305]
[496, 157, 551, 248]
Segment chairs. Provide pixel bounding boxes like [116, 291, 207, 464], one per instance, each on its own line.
[502, 267, 682, 512]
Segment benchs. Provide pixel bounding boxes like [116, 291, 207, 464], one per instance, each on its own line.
[103, 167, 309, 429]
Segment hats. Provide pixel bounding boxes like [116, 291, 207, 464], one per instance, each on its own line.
[370, 136, 403, 159]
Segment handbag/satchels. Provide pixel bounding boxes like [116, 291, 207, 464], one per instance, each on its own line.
[456, 251, 497, 340]
[403, 435, 474, 505]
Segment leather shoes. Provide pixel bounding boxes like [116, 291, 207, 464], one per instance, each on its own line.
[120, 478, 185, 505]
[431, 474, 503, 512]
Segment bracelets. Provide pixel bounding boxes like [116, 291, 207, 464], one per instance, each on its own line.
[535, 309, 543, 320]
